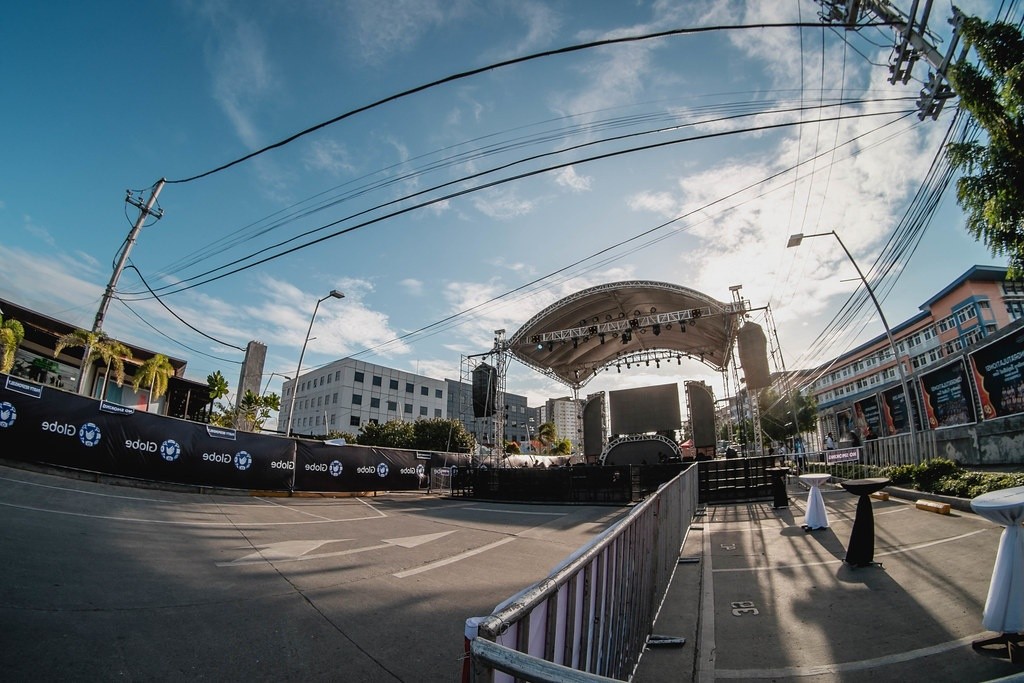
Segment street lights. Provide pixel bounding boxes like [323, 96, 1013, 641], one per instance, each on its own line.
[284, 290, 346, 437]
[786, 229, 923, 472]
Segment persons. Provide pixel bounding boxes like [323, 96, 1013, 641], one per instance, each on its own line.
[658, 447, 739, 465]
[826, 431, 836, 451]
[855, 403, 871, 440]
[771, 437, 808, 473]
[521, 460, 540, 468]
